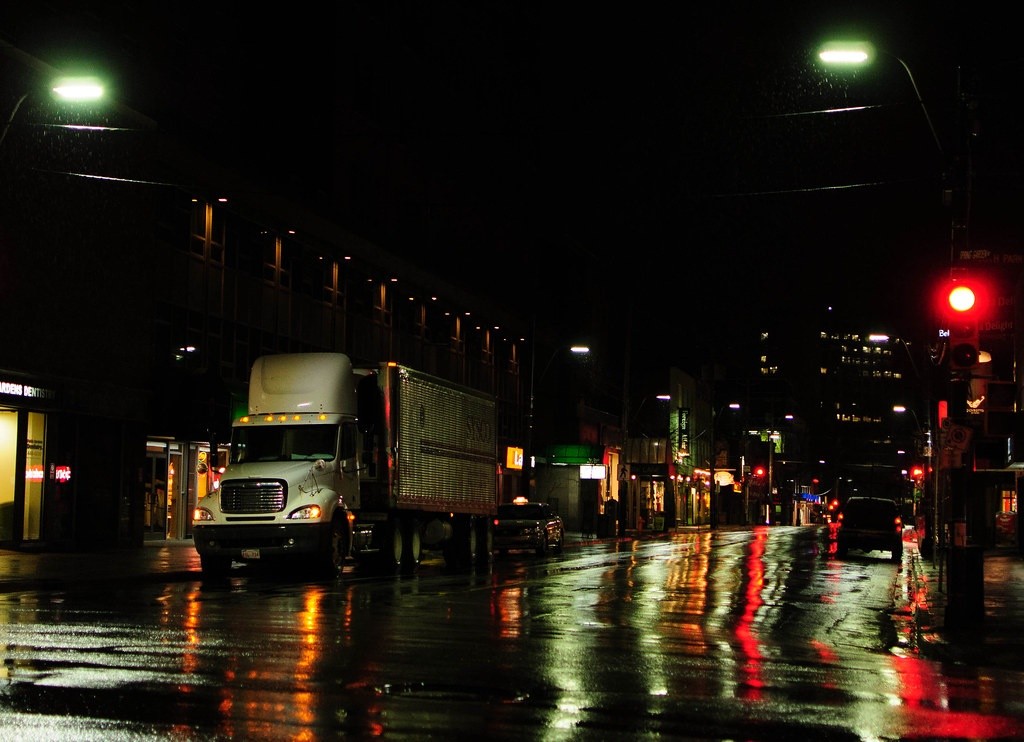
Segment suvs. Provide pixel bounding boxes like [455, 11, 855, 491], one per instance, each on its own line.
[835, 497, 907, 562]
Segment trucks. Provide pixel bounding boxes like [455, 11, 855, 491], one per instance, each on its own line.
[192, 353, 498, 593]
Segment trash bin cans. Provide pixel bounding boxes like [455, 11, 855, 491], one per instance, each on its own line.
[945, 544, 986, 641]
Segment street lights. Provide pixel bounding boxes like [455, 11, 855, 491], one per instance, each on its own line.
[0, 68, 105, 146]
[191, 198, 527, 487]
[812, 37, 964, 566]
[529, 340, 793, 544]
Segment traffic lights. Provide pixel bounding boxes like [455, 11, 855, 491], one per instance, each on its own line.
[944, 266, 981, 367]
[753, 464, 767, 478]
[914, 468, 925, 485]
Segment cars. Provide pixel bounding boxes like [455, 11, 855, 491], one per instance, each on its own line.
[483, 496, 565, 556]
